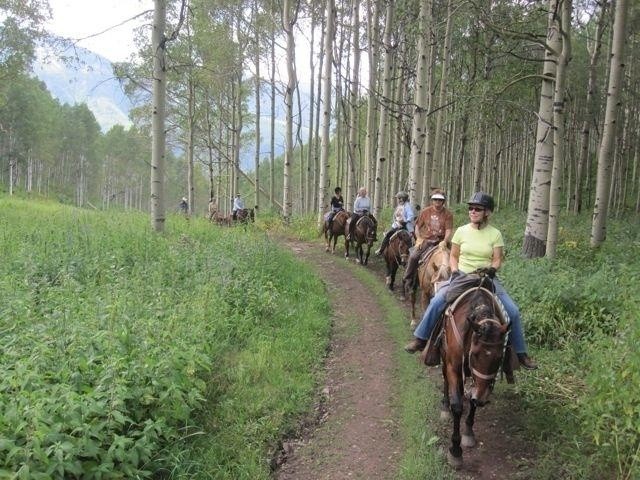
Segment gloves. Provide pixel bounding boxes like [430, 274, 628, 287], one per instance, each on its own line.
[415, 238, 424, 250]
[486, 267, 497, 279]
[450, 271, 460, 283]
[439, 241, 447, 251]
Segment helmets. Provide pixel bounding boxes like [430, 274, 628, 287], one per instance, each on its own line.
[466, 192, 494, 213]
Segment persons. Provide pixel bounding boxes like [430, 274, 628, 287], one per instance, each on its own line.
[208, 197, 217, 219]
[404, 193, 539, 371]
[233, 192, 244, 220]
[179, 196, 188, 213]
[326, 186, 344, 227]
[375, 192, 416, 257]
[402, 189, 453, 286]
[346, 187, 378, 242]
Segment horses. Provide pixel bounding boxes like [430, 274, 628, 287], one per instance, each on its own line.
[344, 214, 377, 266]
[226, 208, 256, 225]
[382, 229, 415, 296]
[206, 211, 226, 226]
[440, 286, 513, 471]
[319, 211, 352, 254]
[400, 246, 451, 327]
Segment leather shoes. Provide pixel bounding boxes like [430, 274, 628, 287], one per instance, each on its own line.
[517, 353, 538, 370]
[403, 338, 426, 354]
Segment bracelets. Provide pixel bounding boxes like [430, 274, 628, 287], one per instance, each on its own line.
[487, 267, 496, 278]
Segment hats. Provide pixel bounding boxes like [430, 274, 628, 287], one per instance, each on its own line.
[395, 191, 408, 199]
[431, 193, 445, 199]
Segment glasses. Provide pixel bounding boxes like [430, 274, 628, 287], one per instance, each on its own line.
[468, 206, 487, 212]
[433, 198, 442, 201]
